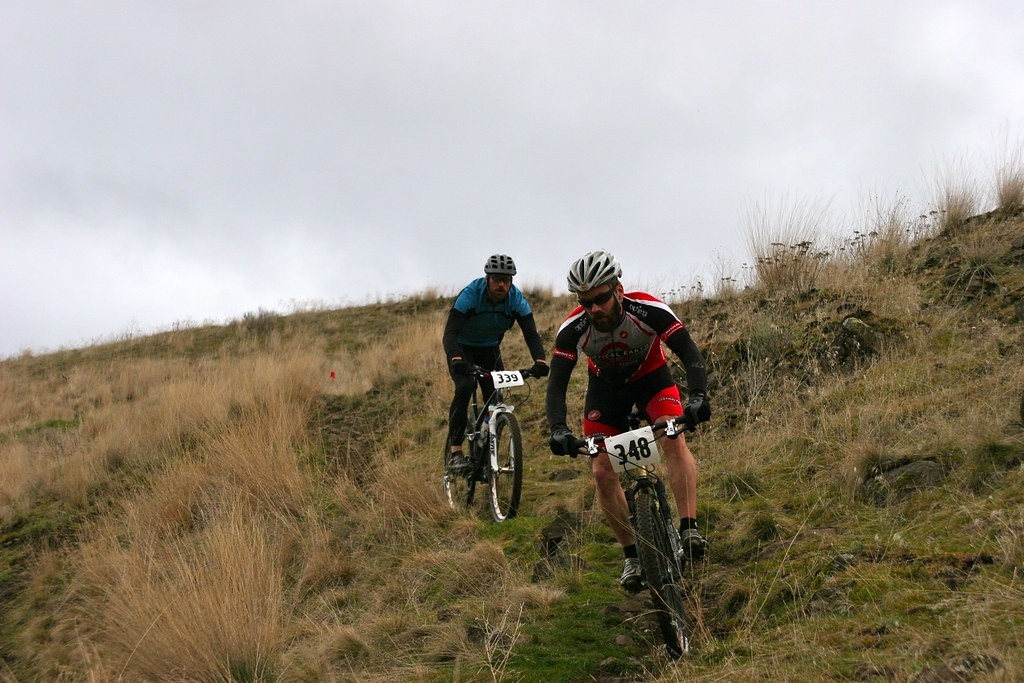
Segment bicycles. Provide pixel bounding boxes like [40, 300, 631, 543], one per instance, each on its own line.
[551, 410, 711, 658]
[443, 364, 535, 523]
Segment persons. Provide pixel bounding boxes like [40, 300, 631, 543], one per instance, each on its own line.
[441, 254, 550, 473]
[543, 249, 712, 592]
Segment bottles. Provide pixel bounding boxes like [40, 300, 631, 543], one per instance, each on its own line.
[477, 416, 490, 448]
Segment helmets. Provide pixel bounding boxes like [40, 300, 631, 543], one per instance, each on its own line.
[483, 254, 517, 276]
[567, 249, 623, 293]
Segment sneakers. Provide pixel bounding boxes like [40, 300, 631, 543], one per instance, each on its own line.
[447, 453, 469, 469]
[483, 463, 500, 482]
[680, 528, 709, 556]
[617, 557, 644, 588]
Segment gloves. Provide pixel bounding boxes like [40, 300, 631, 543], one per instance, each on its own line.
[683, 395, 711, 432]
[549, 428, 578, 458]
[528, 361, 550, 379]
[448, 359, 477, 389]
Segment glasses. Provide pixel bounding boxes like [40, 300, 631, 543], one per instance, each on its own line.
[487, 273, 512, 283]
[577, 283, 619, 308]
